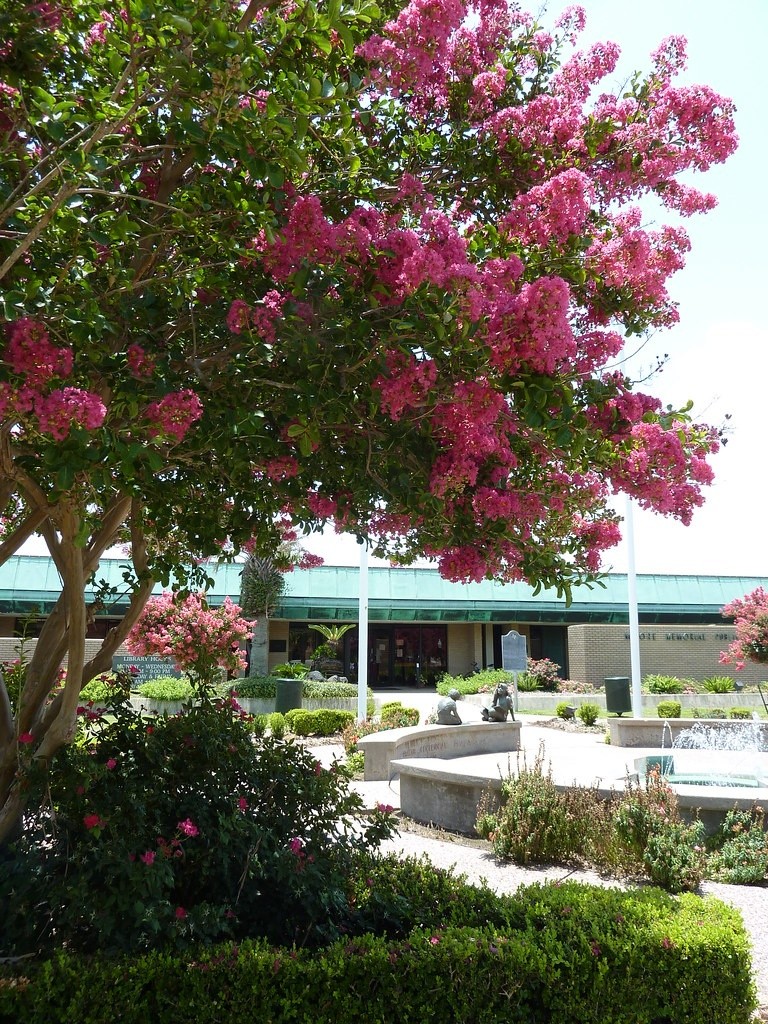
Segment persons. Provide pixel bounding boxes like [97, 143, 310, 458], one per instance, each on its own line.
[435, 688, 462, 725]
[481, 683, 516, 722]
